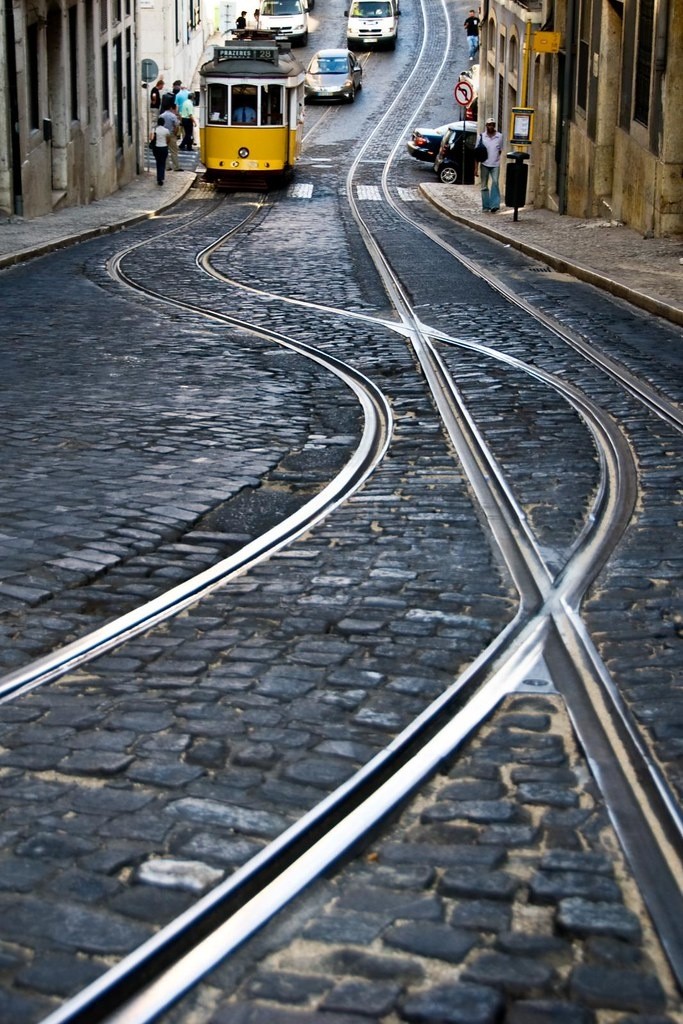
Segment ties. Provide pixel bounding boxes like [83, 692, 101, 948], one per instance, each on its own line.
[242, 108, 245, 122]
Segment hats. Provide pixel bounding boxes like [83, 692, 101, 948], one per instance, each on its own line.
[485, 118, 496, 125]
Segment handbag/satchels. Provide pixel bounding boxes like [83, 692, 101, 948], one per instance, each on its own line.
[148, 128, 156, 148]
[474, 134, 487, 162]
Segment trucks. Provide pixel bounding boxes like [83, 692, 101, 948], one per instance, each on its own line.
[254, 0, 311, 47]
[343, 0, 401, 51]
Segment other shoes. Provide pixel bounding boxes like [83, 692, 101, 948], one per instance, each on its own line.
[158, 181, 162, 186]
[166, 168, 172, 171]
[491, 206, 499, 213]
[469, 56, 473, 60]
[174, 169, 183, 171]
[178, 146, 185, 151]
[482, 208, 488, 212]
[186, 148, 194, 151]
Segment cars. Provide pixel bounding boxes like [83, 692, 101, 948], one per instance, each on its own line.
[434, 121, 477, 184]
[302, 48, 362, 105]
[406, 120, 477, 162]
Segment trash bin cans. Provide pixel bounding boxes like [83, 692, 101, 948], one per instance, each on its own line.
[505, 151, 530, 208]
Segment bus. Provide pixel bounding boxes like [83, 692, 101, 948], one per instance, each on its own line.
[193, 28, 308, 177]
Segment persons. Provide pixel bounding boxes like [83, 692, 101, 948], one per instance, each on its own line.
[317, 62, 330, 72]
[464, 10, 480, 61]
[254, 4, 271, 21]
[158, 103, 184, 172]
[236, 11, 247, 31]
[234, 99, 255, 124]
[381, 3, 391, 14]
[148, 80, 197, 151]
[149, 117, 171, 186]
[473, 117, 504, 213]
